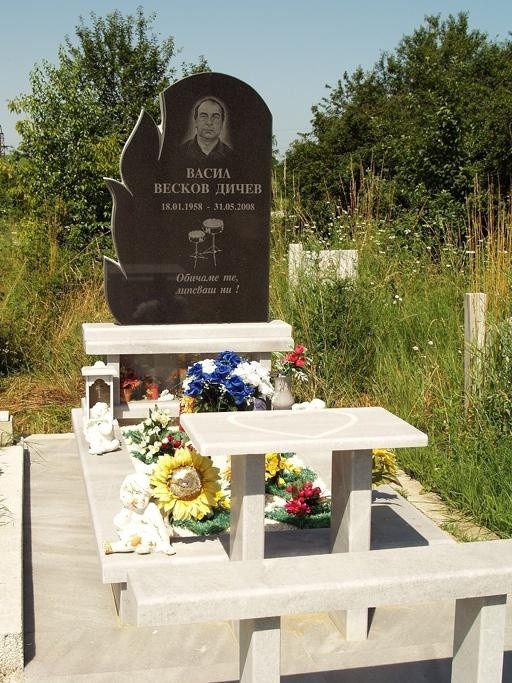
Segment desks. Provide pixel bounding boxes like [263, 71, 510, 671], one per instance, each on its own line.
[179, 406, 429, 644]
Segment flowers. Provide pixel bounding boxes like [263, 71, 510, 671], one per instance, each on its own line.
[129, 344, 402, 533]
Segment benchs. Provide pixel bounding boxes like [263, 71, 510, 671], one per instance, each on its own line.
[128, 540, 512, 683]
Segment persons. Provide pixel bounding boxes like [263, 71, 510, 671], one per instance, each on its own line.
[180, 96, 231, 172]
